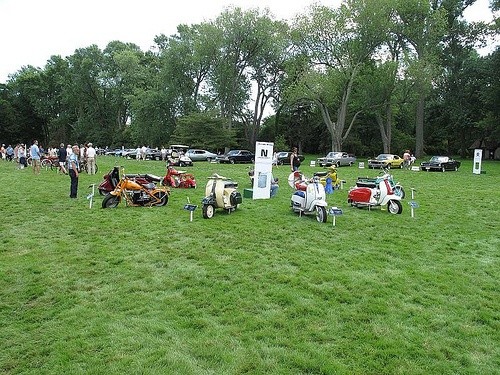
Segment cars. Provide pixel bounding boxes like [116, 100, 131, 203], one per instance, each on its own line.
[215, 149, 256, 165]
[420, 155, 462, 173]
[104, 145, 217, 162]
[367, 153, 405, 169]
[316, 151, 357, 168]
[272, 151, 306, 166]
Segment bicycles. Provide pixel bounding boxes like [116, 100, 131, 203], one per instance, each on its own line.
[27, 153, 99, 175]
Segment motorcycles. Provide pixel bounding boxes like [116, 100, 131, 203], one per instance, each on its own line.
[101, 166, 169, 209]
[98, 166, 161, 197]
[357, 169, 406, 202]
[162, 164, 196, 189]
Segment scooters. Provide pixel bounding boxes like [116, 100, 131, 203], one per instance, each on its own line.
[200, 171, 243, 219]
[287, 170, 330, 224]
[347, 166, 403, 215]
[248, 166, 256, 188]
[312, 167, 342, 191]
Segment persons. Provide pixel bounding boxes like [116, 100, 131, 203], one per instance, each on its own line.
[95, 146, 110, 156]
[86, 143, 96, 175]
[290, 146, 301, 172]
[0, 139, 97, 173]
[57, 143, 69, 175]
[270, 172, 281, 197]
[272, 149, 279, 169]
[120, 144, 168, 161]
[67, 145, 80, 198]
[31, 140, 42, 176]
[403, 148, 416, 171]
